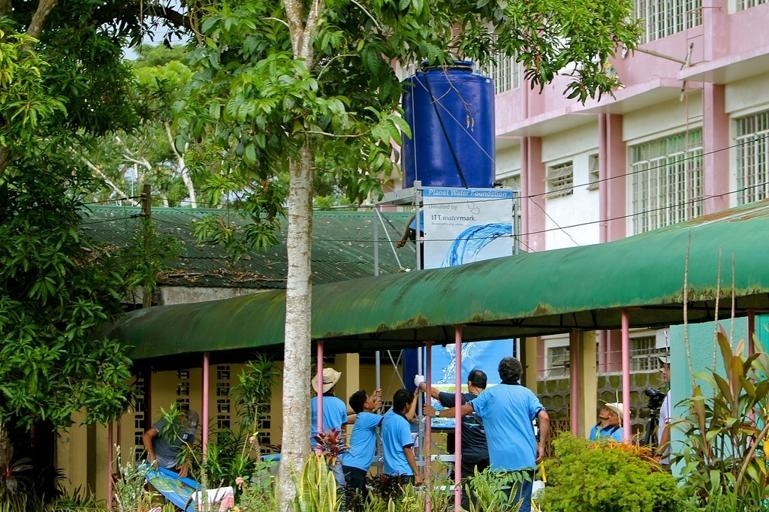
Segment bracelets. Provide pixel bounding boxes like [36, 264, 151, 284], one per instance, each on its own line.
[434, 409, 440, 418]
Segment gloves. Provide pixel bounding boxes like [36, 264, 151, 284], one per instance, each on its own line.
[414, 374, 424, 388]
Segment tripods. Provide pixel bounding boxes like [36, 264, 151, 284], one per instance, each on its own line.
[645, 413, 659, 455]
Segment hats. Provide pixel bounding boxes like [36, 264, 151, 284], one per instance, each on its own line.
[311, 368, 342, 394]
[659, 356, 670, 364]
[183, 410, 198, 434]
[605, 402, 631, 419]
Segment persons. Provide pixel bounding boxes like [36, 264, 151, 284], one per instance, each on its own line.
[589, 401, 613, 440]
[380, 387, 423, 509]
[310, 367, 349, 488]
[143, 410, 199, 504]
[414, 370, 490, 512]
[341, 390, 384, 512]
[395, 206, 424, 270]
[331, 384, 383, 459]
[653, 354, 670, 471]
[423, 357, 550, 512]
[605, 402, 626, 443]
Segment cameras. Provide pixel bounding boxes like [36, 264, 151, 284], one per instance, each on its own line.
[644, 388, 666, 409]
